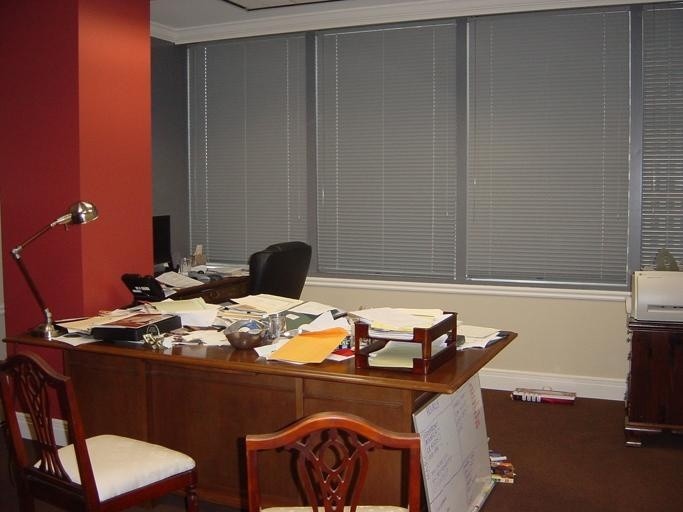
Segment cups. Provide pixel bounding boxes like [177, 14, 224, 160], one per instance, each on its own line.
[178, 257, 192, 277]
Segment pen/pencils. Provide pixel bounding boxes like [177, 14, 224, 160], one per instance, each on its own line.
[229, 310, 257, 315]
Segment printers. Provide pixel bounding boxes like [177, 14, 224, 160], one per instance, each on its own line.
[631, 271, 683, 323]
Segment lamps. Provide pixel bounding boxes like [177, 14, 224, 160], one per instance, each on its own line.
[11, 202, 100, 337]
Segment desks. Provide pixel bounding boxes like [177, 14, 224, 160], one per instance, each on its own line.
[623, 316, 683, 447]
[158, 263, 250, 302]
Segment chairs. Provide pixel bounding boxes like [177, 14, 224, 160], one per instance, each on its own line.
[239, 412, 420, 512]
[0, 353, 199, 512]
[249, 242, 313, 300]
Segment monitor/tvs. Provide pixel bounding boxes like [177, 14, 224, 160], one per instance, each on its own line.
[153, 215, 170, 265]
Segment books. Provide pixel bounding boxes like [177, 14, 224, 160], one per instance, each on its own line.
[511, 386, 578, 404]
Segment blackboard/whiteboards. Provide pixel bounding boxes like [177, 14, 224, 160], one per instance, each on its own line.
[412, 374, 495, 512]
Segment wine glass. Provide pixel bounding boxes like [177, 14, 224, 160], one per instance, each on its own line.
[266, 314, 288, 353]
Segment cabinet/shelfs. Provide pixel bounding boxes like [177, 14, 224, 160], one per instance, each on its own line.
[0, 301, 516, 511]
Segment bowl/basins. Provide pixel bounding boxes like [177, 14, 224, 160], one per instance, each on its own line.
[223, 318, 267, 351]
